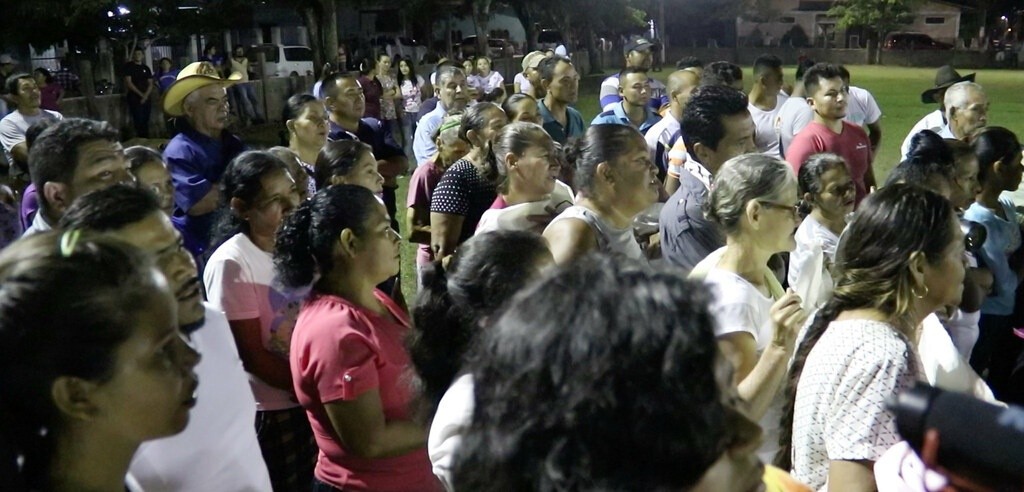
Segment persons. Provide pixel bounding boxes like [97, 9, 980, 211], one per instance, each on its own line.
[158, 59, 264, 258]
[451, 261, 772, 492]
[0, 71, 70, 188]
[784, 61, 877, 209]
[271, 183, 439, 490]
[684, 151, 812, 466]
[0, 227, 204, 492]
[539, 123, 661, 259]
[787, 183, 972, 490]
[0, 37, 1024, 491]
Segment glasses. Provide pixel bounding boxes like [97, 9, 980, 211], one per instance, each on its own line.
[759, 200, 805, 219]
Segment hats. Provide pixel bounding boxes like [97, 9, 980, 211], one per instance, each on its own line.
[159, 62, 243, 118]
[921, 66, 975, 103]
[623, 34, 654, 55]
[523, 50, 548, 73]
